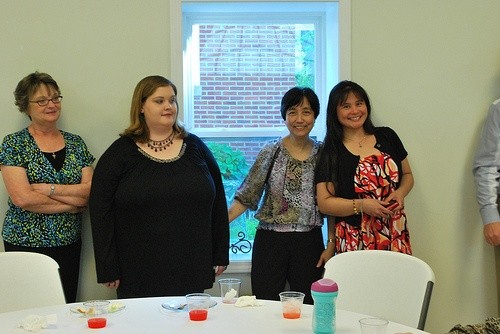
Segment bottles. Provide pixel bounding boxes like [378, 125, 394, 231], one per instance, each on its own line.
[310, 278, 339, 334]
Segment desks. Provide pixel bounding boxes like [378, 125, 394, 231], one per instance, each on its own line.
[0, 296, 430, 334]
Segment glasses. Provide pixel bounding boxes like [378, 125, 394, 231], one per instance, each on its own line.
[29, 96, 63, 106]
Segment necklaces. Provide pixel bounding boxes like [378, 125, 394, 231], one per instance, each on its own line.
[344, 133, 366, 148]
[146, 131, 175, 152]
[30, 127, 57, 159]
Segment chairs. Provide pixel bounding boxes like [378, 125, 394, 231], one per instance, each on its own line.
[323, 249, 436, 331]
[0, 252, 66, 310]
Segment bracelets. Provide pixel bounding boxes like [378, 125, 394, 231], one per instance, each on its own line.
[353, 199, 359, 215]
[361, 199, 363, 215]
[49, 183, 56, 197]
[327, 239, 334, 242]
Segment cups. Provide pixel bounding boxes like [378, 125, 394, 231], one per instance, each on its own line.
[219, 278, 241, 304]
[186, 293, 211, 321]
[279, 291, 305, 319]
[359, 317, 390, 334]
[83, 300, 109, 328]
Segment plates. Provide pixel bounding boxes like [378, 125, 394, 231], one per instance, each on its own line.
[161, 298, 217, 312]
[69, 304, 125, 314]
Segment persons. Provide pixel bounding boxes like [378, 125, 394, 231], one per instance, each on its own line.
[314, 80, 414, 256]
[228, 88, 335, 305]
[89, 75, 231, 299]
[0, 71, 96, 304]
[471, 98, 500, 246]
